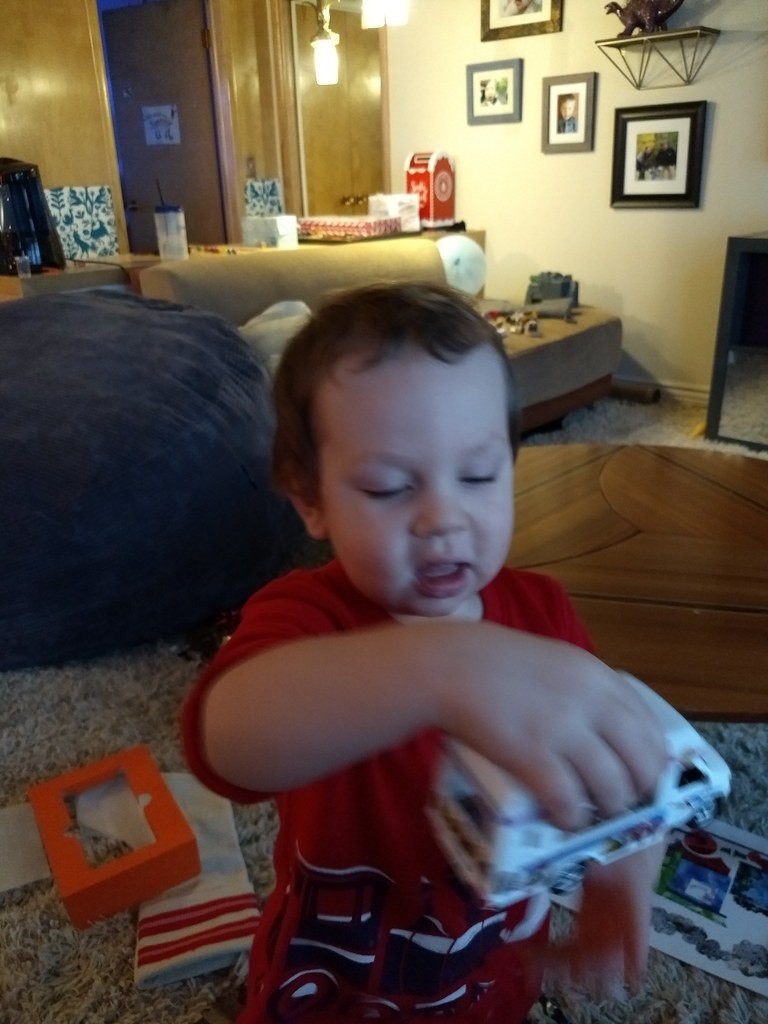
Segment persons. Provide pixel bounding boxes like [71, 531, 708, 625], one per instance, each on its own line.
[503, 0, 538, 15]
[558, 94, 578, 133]
[181, 281, 667, 1024]
[481, 79, 499, 108]
[636, 141, 677, 180]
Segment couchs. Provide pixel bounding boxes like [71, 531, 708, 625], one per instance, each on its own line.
[140, 236, 622, 439]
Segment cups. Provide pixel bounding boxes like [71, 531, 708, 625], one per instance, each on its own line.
[154, 204, 189, 262]
[15, 256, 32, 280]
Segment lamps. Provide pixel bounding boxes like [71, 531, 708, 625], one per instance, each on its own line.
[303, 0, 410, 85]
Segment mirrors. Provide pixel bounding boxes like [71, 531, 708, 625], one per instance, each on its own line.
[705, 236, 768, 453]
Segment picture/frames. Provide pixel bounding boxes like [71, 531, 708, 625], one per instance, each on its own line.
[466, 58, 523, 126]
[541, 72, 597, 154]
[481, 0, 563, 42]
[611, 99, 708, 209]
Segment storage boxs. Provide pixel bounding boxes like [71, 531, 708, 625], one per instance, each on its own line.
[240, 216, 298, 249]
[24, 746, 204, 927]
[369, 195, 420, 234]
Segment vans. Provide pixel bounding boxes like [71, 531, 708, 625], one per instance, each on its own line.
[421, 670, 734, 913]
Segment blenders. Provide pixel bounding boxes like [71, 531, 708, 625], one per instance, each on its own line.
[0, 157, 48, 274]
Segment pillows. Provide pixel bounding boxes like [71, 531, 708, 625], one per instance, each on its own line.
[234, 302, 313, 378]
